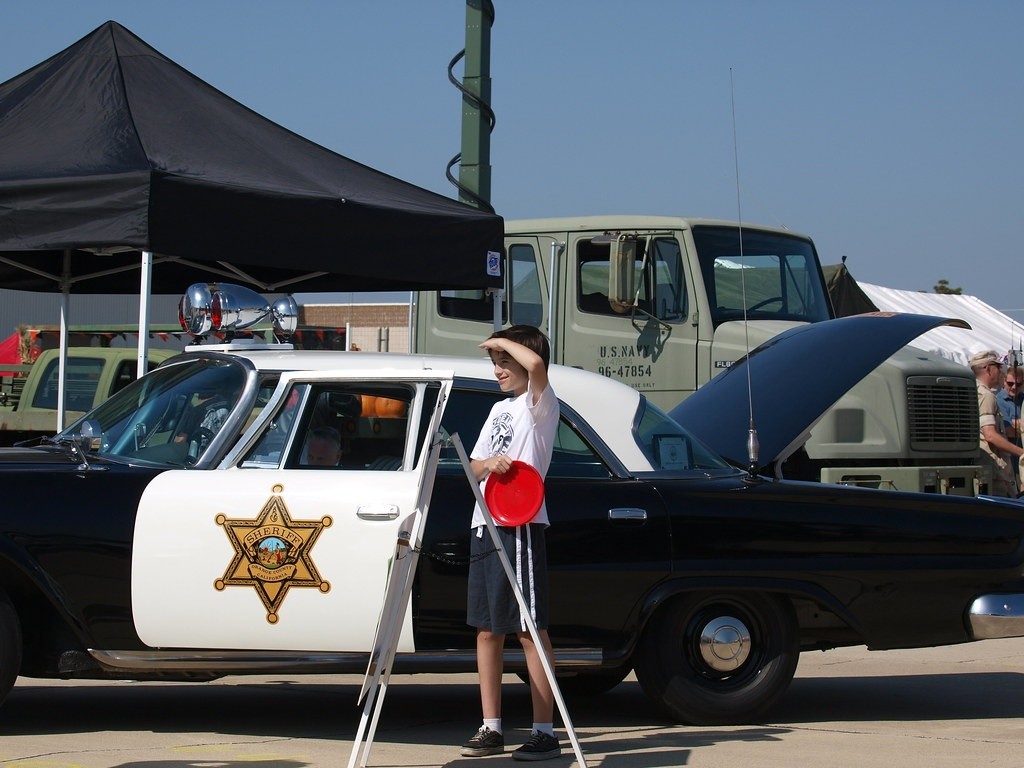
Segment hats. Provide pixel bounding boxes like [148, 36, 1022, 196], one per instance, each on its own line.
[970, 350, 1004, 369]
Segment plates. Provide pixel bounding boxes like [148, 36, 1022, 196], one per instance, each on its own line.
[484, 460, 544, 527]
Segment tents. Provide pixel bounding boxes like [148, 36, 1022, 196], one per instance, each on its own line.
[714, 257, 1024, 367]
[0, 21, 506, 439]
[500, 254, 881, 323]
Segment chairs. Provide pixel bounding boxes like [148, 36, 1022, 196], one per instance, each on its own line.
[366, 454, 402, 471]
[581, 292, 614, 314]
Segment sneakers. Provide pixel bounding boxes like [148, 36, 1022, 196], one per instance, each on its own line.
[512, 729, 561, 760]
[460, 725, 505, 756]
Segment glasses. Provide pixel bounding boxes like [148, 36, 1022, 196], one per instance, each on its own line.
[1005, 380, 1024, 387]
[983, 364, 1001, 370]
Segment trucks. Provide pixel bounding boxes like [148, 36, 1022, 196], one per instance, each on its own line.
[413, 215, 991, 501]
[0, 343, 285, 461]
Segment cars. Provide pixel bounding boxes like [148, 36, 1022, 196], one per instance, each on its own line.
[0, 283, 1024, 727]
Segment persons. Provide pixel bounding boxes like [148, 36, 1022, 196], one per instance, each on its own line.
[968, 350, 1024, 498]
[462, 325, 562, 759]
[175, 391, 233, 465]
[306, 426, 341, 466]
